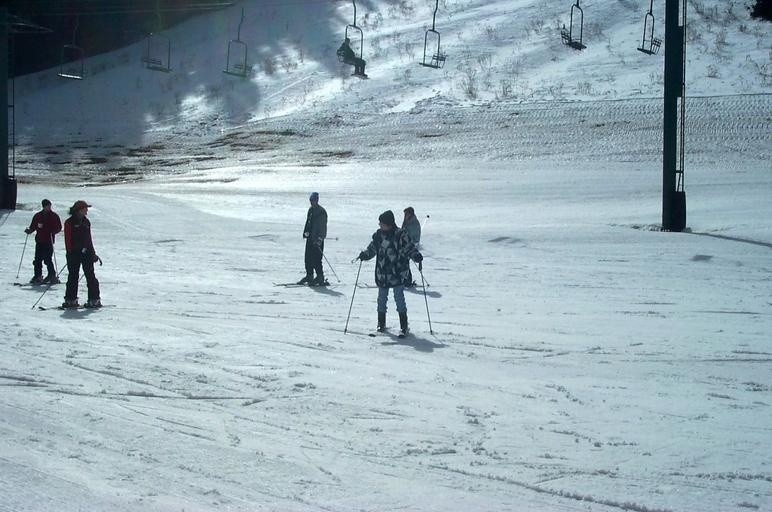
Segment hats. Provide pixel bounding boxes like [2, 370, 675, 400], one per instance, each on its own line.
[309, 192, 319, 202]
[74, 200, 92, 210]
[379, 209, 395, 225]
[42, 199, 51, 206]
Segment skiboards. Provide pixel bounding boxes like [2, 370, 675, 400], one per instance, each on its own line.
[40, 303, 116, 310]
[272, 280, 340, 289]
[369, 327, 411, 339]
[13, 280, 68, 289]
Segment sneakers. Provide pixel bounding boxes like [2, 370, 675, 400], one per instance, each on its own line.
[30, 275, 57, 283]
[62, 298, 78, 307]
[299, 274, 324, 285]
[86, 299, 101, 307]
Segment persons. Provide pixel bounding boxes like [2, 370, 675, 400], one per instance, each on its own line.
[23, 198, 62, 285]
[336, 37, 369, 78]
[358, 210, 423, 334]
[63, 200, 104, 308]
[401, 206, 421, 284]
[296, 191, 328, 285]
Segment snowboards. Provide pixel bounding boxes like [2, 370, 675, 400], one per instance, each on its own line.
[351, 73, 367, 80]
